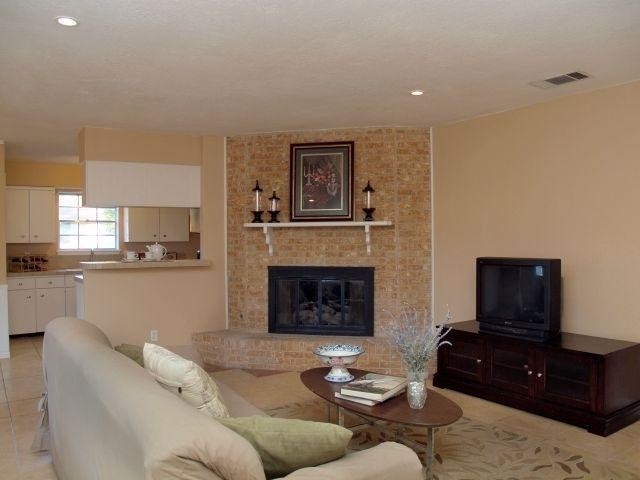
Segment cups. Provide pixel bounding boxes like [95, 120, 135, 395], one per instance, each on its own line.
[124, 250, 155, 258]
[197, 250, 200, 259]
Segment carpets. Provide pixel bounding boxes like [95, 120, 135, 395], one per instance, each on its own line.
[256, 396, 640, 480]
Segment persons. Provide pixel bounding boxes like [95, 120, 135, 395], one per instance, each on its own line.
[326, 173, 340, 207]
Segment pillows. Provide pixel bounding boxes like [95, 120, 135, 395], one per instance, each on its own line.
[113, 343, 145, 369]
[141, 340, 229, 421]
[212, 414, 353, 479]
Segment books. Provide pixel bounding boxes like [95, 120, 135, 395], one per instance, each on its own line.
[340, 370, 408, 403]
[335, 389, 407, 406]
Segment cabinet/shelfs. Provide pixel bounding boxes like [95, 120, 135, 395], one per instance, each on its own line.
[5, 188, 54, 244]
[66, 276, 77, 318]
[121, 206, 189, 243]
[432, 318, 640, 438]
[7, 276, 66, 335]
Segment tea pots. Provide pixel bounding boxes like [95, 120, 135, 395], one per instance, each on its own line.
[145, 241, 168, 260]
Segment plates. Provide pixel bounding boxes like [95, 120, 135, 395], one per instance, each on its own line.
[120, 256, 156, 263]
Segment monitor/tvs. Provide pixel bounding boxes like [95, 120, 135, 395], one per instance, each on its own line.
[475, 257, 561, 342]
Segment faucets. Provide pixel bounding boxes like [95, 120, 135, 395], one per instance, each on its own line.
[90, 248, 95, 261]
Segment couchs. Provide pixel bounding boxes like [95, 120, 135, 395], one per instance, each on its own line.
[29, 317, 427, 479]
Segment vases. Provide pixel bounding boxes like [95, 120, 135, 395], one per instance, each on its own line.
[406, 369, 428, 410]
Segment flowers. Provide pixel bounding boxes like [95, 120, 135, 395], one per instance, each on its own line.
[365, 302, 455, 380]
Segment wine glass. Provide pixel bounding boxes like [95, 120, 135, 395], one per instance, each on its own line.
[312, 342, 366, 384]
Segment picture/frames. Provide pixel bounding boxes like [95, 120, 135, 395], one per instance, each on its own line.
[288, 140, 355, 221]
